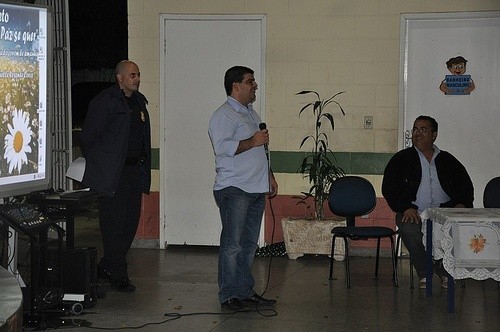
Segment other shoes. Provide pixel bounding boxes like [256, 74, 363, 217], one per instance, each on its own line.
[240, 293, 277, 306]
[433, 268, 448, 289]
[221, 298, 250, 311]
[98, 259, 136, 293]
[418, 278, 427, 289]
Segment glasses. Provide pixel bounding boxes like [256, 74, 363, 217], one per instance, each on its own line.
[411, 127, 432, 135]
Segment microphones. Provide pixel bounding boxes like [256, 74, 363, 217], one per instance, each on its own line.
[259, 122, 268, 154]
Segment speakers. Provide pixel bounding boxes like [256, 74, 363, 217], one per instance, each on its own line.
[32, 244, 98, 308]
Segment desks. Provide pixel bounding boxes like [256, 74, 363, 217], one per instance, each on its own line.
[40, 193, 105, 245]
[422, 208, 500, 313]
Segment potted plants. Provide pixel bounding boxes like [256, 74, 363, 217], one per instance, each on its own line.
[280, 90, 348, 262]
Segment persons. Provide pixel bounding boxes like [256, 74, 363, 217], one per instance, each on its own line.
[80, 60, 151, 293]
[382, 114, 474, 289]
[208, 66, 279, 309]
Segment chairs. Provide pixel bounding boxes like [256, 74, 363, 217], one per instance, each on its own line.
[328, 176, 399, 288]
[394, 230, 416, 290]
[483, 177, 500, 208]
